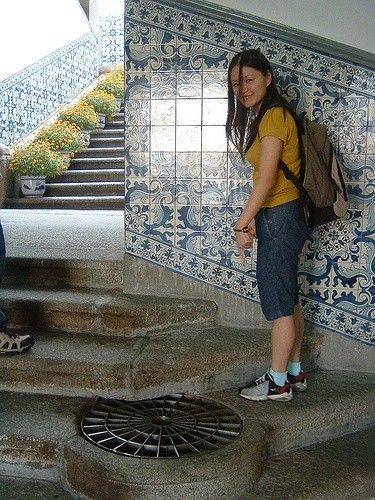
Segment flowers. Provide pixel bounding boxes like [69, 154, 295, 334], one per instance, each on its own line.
[11, 64, 127, 175]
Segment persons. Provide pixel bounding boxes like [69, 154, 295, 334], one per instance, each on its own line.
[225, 50, 307, 402]
[0, 223, 35, 354]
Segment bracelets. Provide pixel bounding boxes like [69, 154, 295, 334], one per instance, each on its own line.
[233, 226, 250, 233]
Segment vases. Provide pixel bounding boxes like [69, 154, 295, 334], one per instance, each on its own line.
[19, 97, 124, 198]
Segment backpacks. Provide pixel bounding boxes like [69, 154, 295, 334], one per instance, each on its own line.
[259, 104, 349, 228]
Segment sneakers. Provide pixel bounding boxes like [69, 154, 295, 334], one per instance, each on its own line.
[288, 369, 307, 391]
[238, 371, 293, 402]
[0, 326, 34, 356]
